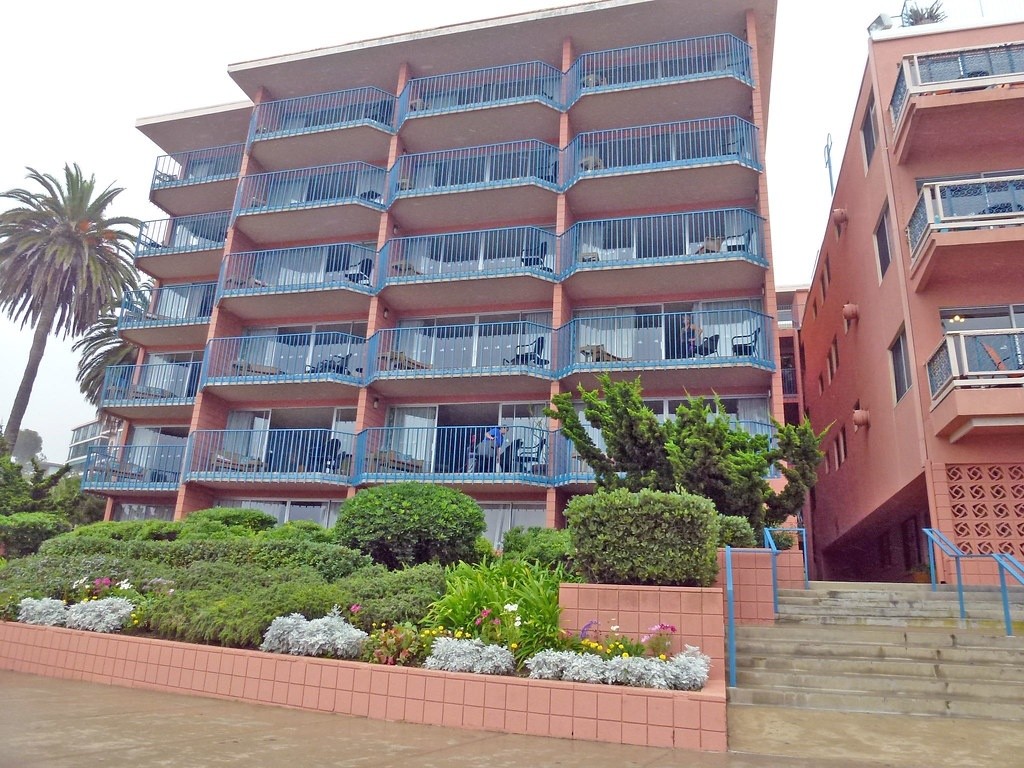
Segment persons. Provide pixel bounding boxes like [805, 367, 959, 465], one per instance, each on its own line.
[680, 315, 703, 359]
[465, 434, 479, 472]
[477, 424, 509, 473]
